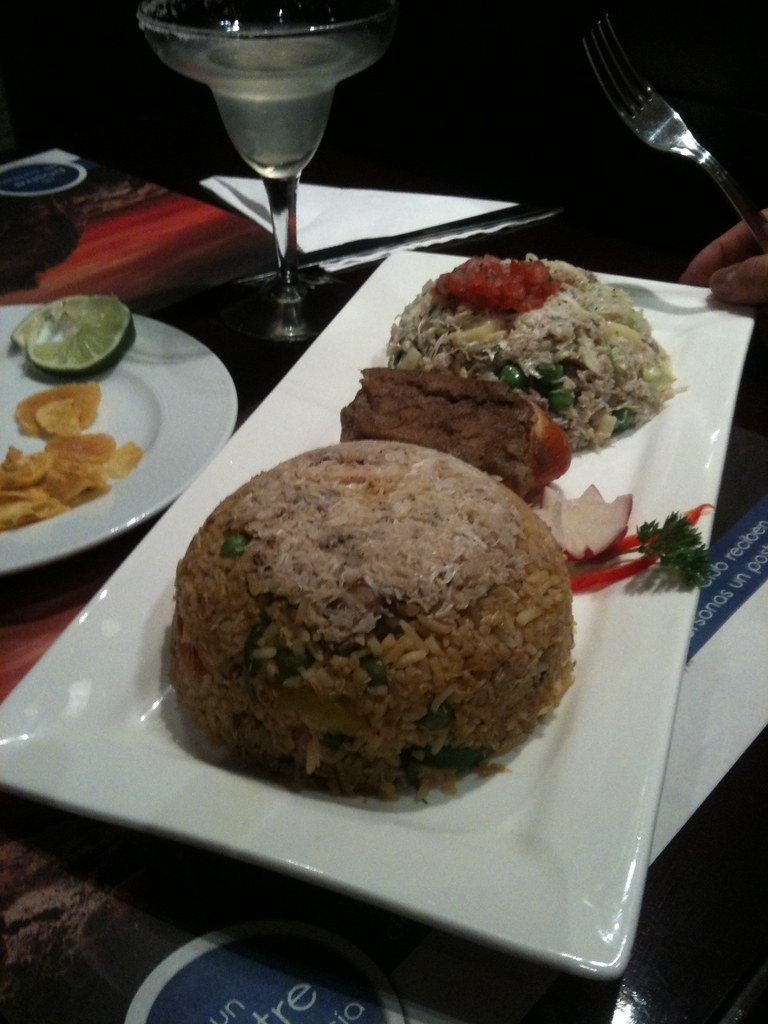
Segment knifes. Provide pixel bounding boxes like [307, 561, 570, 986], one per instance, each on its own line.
[167, 200, 565, 294]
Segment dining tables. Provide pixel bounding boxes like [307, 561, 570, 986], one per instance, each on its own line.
[1, 132, 766, 1024]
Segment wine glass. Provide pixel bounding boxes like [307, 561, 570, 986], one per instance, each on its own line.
[138, 0, 399, 343]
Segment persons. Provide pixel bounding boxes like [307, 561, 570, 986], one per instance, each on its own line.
[676, 201, 768, 327]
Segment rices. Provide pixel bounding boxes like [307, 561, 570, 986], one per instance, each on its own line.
[171, 255, 685, 808]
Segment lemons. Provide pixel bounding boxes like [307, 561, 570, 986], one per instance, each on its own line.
[8, 291, 138, 380]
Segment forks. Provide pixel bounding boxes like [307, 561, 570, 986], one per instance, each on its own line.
[581, 12, 766, 259]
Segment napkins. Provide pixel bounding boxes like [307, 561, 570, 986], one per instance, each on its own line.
[194, 166, 562, 276]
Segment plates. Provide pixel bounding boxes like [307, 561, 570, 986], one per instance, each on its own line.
[1, 249, 754, 981]
[2, 303, 240, 576]
